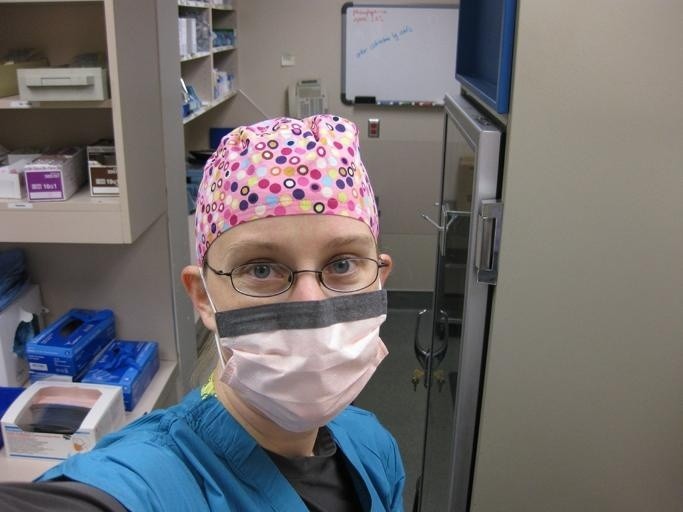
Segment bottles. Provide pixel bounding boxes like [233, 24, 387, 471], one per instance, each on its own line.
[193, 113, 381, 266]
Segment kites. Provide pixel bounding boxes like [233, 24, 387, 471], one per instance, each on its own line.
[378, 101, 436, 106]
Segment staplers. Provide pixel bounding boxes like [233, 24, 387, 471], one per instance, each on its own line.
[355, 96, 375, 104]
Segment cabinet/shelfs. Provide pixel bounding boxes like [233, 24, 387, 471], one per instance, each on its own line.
[175, 2, 239, 393]
[1, 360, 179, 485]
[1, 2, 164, 244]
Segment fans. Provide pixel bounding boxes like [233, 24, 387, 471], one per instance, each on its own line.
[341, 2, 461, 107]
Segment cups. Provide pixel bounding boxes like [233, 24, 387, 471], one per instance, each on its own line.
[202, 256, 388, 299]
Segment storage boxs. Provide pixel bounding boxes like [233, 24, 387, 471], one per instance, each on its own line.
[82, 338, 160, 411]
[23, 147, 86, 200]
[27, 309, 118, 383]
[1, 380, 126, 461]
[87, 140, 120, 197]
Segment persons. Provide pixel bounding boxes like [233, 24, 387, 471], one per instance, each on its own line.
[0, 112, 407, 510]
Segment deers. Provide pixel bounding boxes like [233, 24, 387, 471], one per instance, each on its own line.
[200, 268, 392, 434]
